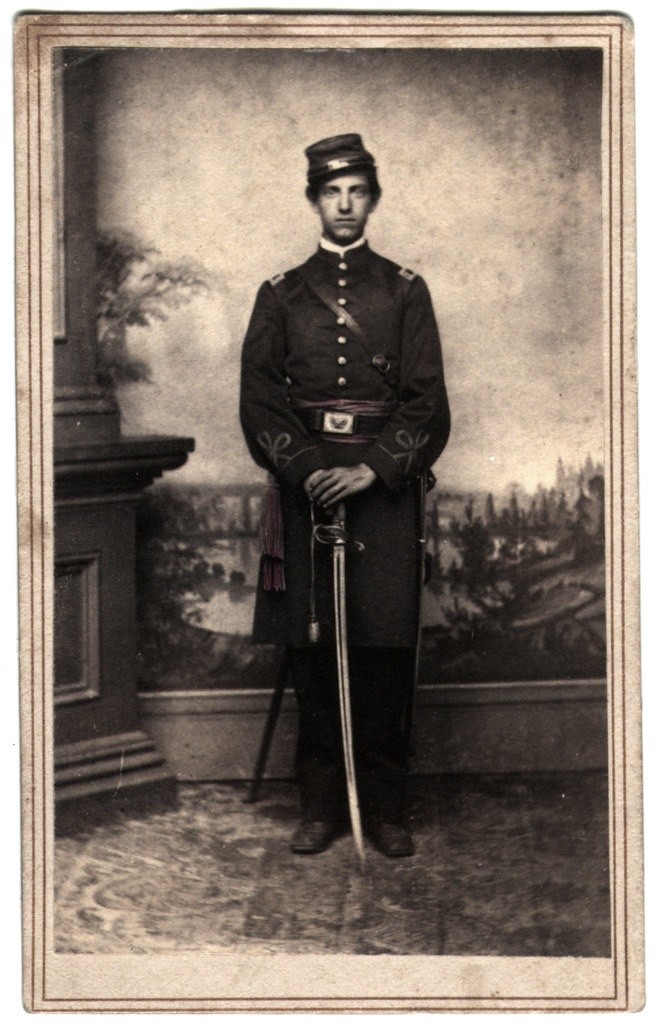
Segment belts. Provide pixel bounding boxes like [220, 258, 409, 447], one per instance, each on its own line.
[293, 407, 387, 436]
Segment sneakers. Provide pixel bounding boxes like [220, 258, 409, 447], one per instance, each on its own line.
[368, 820, 415, 859]
[288, 819, 346, 854]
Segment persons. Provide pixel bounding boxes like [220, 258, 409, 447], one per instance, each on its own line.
[239, 133, 452, 857]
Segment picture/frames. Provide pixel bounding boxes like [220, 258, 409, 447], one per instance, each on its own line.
[10, 9, 648, 1017]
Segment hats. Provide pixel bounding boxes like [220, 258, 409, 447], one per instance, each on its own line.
[305, 133, 375, 180]
